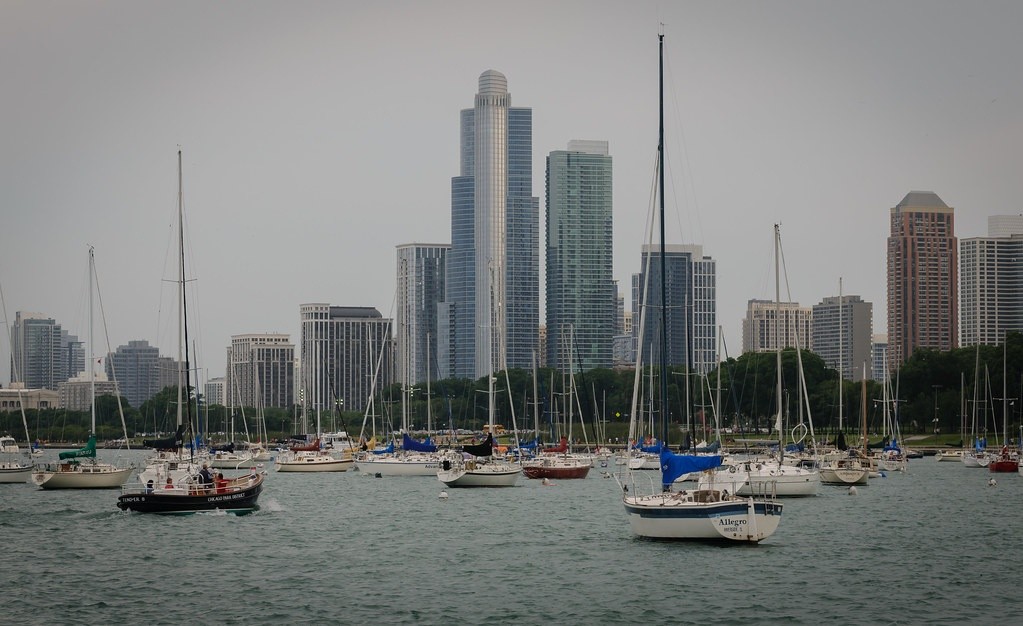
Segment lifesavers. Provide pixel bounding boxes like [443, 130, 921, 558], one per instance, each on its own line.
[273, 464, 281, 472]
[282, 456, 288, 464]
[729, 466, 735, 474]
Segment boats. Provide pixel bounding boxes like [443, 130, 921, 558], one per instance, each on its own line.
[22, 446, 43, 457]
[0, 436, 20, 454]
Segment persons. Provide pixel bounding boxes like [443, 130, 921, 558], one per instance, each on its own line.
[145, 480, 154, 494]
[216, 473, 227, 493]
[197, 465, 213, 495]
[165, 477, 174, 489]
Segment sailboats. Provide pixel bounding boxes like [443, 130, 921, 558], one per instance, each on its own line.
[934, 328, 1023, 472]
[0, 283, 36, 484]
[434, 267, 525, 488]
[615, 32, 786, 547]
[521, 323, 594, 480]
[29, 246, 137, 490]
[620, 218, 921, 498]
[138, 185, 574, 475]
[114, 147, 272, 519]
